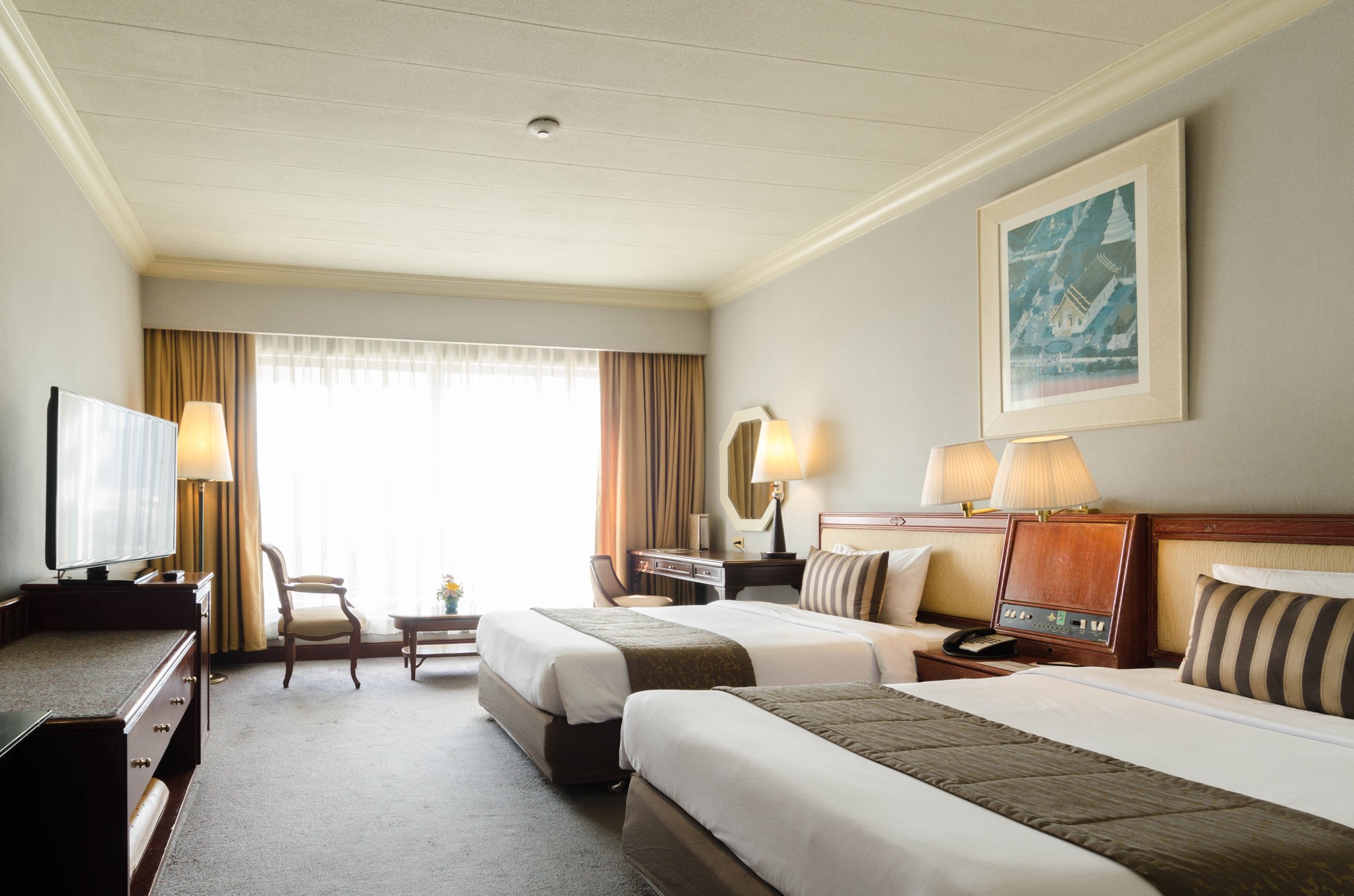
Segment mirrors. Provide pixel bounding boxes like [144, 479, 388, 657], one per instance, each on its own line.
[717, 405, 784, 532]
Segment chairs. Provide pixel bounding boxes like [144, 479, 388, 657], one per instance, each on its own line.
[259, 542, 368, 690]
[589, 554, 675, 610]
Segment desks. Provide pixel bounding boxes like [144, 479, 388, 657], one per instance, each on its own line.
[628, 546, 809, 606]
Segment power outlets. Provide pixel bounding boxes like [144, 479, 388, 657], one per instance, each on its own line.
[732, 537, 744, 548]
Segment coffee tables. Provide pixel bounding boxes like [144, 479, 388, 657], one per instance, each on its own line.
[384, 608, 484, 682]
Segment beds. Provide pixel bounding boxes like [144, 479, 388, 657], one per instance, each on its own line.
[615, 514, 1354, 896]
[472, 510, 1015, 796]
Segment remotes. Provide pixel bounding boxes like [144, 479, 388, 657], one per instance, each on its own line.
[162, 570, 185, 580]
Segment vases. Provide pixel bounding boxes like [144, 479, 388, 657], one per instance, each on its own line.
[445, 600, 459, 611]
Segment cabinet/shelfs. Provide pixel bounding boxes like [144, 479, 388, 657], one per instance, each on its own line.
[2, 568, 213, 896]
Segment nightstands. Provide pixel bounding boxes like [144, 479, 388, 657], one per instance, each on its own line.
[912, 646, 1055, 679]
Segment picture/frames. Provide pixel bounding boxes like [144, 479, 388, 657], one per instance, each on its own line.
[971, 116, 1192, 441]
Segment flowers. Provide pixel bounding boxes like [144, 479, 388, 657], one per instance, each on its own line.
[435, 573, 464, 608]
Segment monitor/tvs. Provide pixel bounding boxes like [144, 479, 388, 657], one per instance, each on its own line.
[47, 385, 178, 586]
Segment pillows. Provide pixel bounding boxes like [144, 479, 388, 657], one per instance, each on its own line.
[1212, 563, 1354, 600]
[798, 544, 889, 625]
[1174, 573, 1354, 723]
[833, 542, 934, 628]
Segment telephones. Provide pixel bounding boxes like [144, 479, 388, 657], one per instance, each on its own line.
[942, 627, 1017, 659]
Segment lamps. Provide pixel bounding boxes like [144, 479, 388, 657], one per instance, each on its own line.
[176, 401, 236, 684]
[920, 439, 1004, 519]
[989, 433, 1103, 523]
[749, 418, 805, 559]
